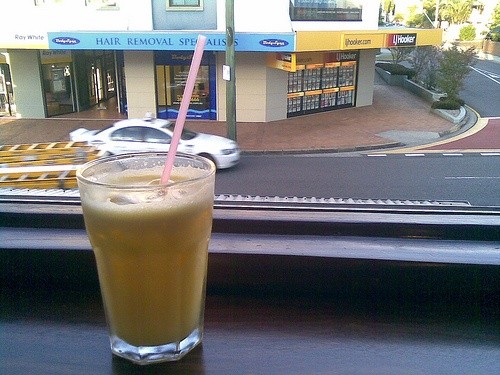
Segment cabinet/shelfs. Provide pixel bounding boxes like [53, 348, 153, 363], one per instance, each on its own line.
[287, 65, 355, 113]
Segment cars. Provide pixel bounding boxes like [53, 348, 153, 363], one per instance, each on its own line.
[70, 117, 241, 171]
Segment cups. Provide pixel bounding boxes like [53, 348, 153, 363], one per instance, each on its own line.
[75, 150, 215, 367]
